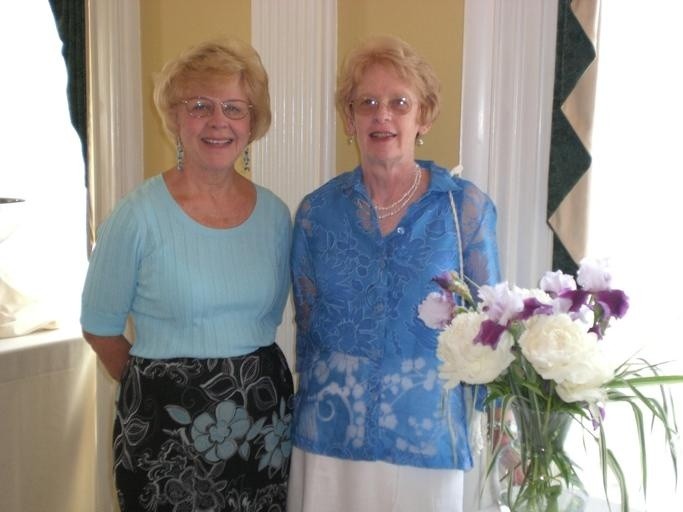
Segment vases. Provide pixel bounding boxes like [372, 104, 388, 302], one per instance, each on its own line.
[492, 400, 597, 511]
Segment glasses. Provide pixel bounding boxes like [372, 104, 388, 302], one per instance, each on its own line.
[353, 94, 412, 114]
[180, 96, 252, 120]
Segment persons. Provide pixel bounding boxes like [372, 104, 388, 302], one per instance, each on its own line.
[80, 37, 293, 512]
[286, 37, 528, 512]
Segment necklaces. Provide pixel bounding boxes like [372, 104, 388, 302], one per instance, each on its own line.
[356, 164, 421, 220]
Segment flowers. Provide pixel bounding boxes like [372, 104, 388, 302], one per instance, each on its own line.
[418, 265, 683, 510]
